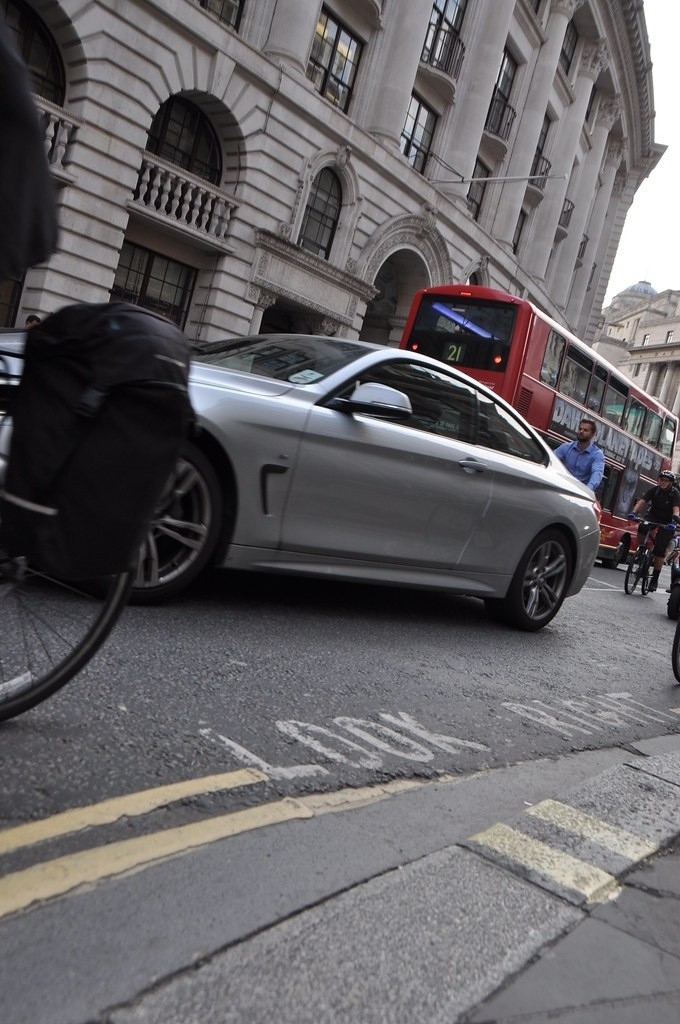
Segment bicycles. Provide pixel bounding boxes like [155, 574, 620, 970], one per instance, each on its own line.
[624, 515, 674, 596]
[0, 307, 152, 722]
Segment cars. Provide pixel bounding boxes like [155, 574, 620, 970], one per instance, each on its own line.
[1, 330, 604, 636]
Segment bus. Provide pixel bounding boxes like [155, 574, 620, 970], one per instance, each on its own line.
[397, 284, 680, 569]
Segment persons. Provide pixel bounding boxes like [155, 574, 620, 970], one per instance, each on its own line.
[25, 314, 41, 329]
[553, 419, 605, 493]
[626, 470, 680, 591]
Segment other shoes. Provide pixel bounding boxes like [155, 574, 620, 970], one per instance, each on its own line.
[626, 556, 642, 564]
[648, 578, 658, 592]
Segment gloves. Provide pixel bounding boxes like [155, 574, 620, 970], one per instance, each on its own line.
[627, 512, 636, 520]
[667, 522, 677, 531]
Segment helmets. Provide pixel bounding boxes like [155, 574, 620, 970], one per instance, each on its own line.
[659, 470, 676, 484]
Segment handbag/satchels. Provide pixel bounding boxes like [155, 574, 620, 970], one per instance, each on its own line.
[0, 301, 198, 605]
